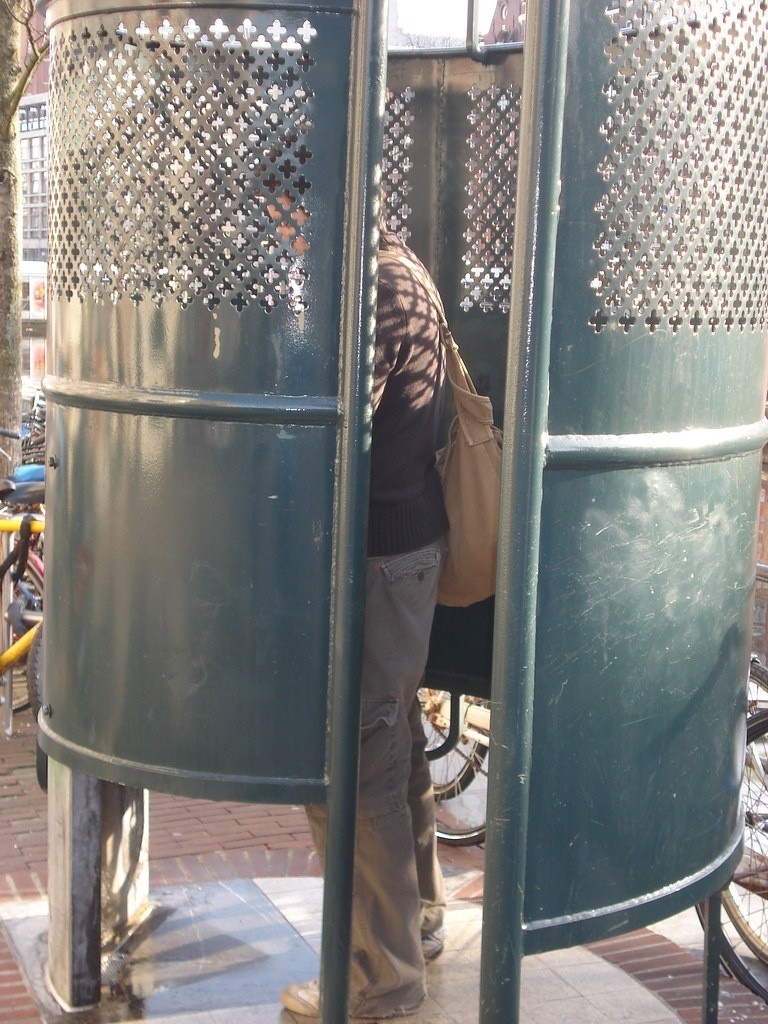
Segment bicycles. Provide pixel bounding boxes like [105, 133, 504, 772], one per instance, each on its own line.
[417, 563, 768, 1002]
[0, 396, 48, 720]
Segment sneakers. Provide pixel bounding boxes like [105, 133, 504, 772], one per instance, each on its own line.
[282, 976, 428, 1019]
[420, 926, 447, 963]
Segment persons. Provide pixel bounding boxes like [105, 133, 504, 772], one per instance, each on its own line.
[254, 130, 452, 1018]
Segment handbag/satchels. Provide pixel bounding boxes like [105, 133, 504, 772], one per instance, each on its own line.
[434, 431, 503, 606]
